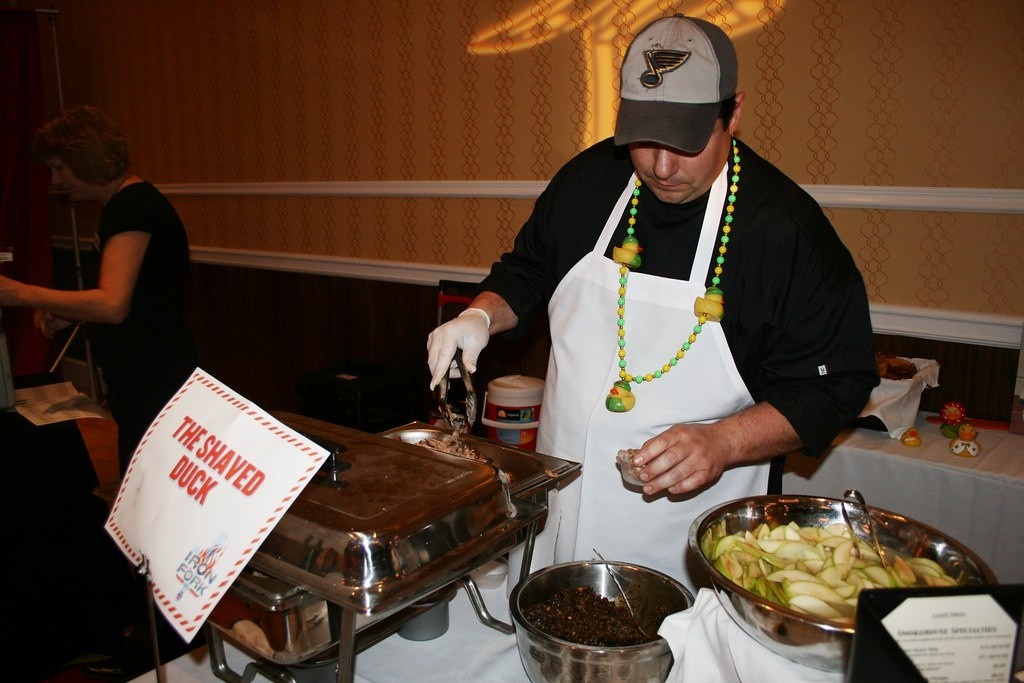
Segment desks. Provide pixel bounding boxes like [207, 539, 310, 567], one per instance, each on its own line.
[129, 558, 536, 682]
[782, 410, 1024, 587]
[0, 383, 114, 681]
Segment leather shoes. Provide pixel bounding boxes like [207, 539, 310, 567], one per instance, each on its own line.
[83, 619, 178, 679]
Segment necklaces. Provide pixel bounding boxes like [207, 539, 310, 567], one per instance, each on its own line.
[606, 133, 742, 410]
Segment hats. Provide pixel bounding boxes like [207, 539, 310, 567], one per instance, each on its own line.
[614, 12, 739, 153]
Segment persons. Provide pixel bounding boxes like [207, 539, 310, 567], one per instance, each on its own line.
[0, 105, 201, 681]
[427, 14, 880, 596]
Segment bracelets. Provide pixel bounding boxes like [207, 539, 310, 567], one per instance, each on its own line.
[458, 308, 491, 327]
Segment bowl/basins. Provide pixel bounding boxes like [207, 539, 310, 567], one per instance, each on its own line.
[618, 449, 648, 487]
[469, 557, 509, 591]
[688, 494, 997, 675]
[509, 560, 696, 683]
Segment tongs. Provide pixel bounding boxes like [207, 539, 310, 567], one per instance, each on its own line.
[841, 488, 885, 567]
[438, 351, 476, 431]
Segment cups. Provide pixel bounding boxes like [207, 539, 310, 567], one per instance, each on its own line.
[396, 589, 457, 641]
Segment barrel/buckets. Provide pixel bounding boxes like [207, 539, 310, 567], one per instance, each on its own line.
[481, 375, 545, 453]
